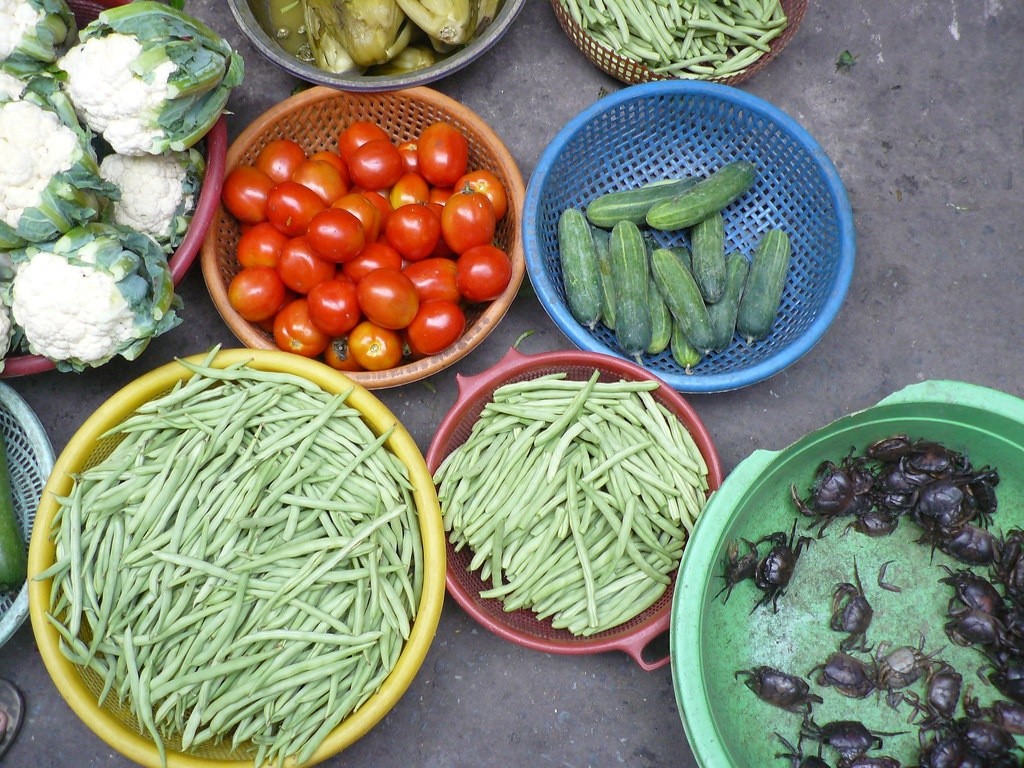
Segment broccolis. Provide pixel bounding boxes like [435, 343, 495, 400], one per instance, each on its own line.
[0, 0, 245, 369]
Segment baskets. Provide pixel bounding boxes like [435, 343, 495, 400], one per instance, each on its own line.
[1, 1, 229, 376]
[552, 0, 810, 84]
[29, 349, 446, 768]
[201, 84, 526, 391]
[523, 79, 856, 392]
[0, 384, 57, 648]
[426, 345, 724, 671]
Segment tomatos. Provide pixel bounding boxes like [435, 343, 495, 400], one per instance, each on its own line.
[221, 121, 512, 371]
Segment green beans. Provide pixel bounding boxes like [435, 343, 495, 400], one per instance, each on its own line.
[433, 368, 710, 637]
[31, 339, 426, 768]
[559, 0, 792, 79]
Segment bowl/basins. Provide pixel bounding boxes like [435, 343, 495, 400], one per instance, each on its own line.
[669, 378, 1024, 768]
[228, 0, 524, 92]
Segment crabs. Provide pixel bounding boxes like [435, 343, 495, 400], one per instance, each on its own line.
[711, 432, 1024, 768]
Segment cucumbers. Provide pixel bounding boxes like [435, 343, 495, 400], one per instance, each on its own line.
[557, 162, 790, 369]
